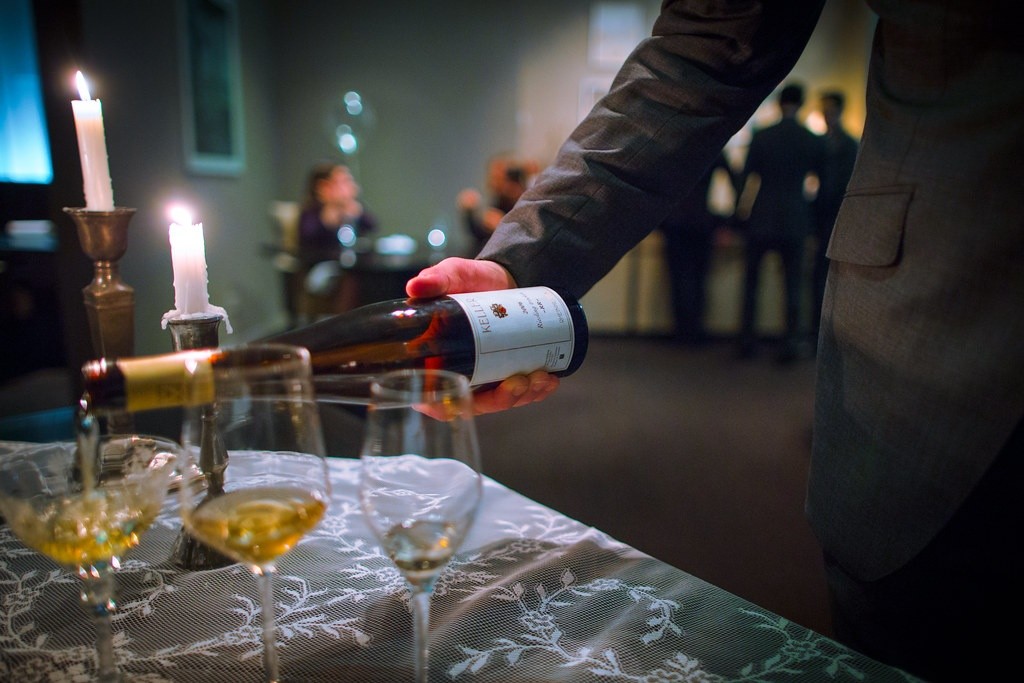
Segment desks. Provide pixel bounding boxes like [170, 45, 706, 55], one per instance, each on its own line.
[1, 441, 928, 683]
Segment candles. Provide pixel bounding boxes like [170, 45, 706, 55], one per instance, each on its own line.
[168, 202, 209, 316]
[71, 70, 115, 211]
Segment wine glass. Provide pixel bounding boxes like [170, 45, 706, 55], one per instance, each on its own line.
[0, 406, 181, 683]
[182, 342, 329, 683]
[359, 369, 483, 683]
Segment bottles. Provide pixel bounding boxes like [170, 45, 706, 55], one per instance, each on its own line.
[83, 285, 589, 414]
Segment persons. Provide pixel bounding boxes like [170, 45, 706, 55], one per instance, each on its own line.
[288, 159, 374, 327]
[731, 78, 831, 366]
[410, 0, 1024, 683]
[810, 88, 860, 353]
[654, 150, 737, 348]
[456, 156, 539, 241]
